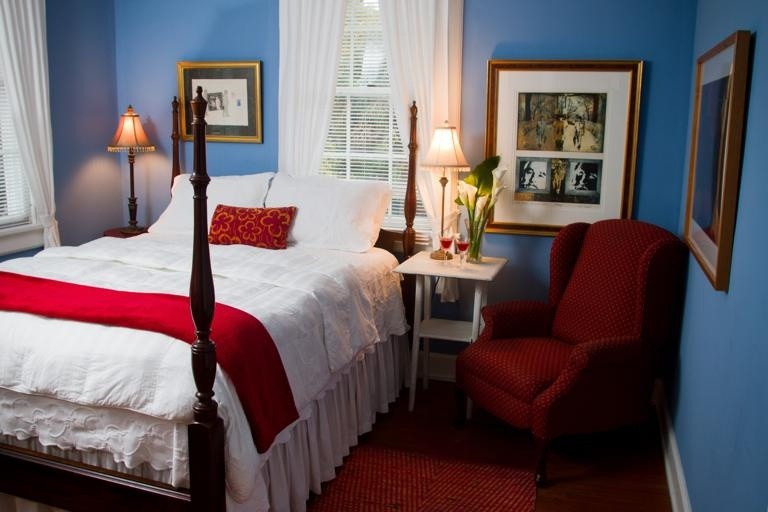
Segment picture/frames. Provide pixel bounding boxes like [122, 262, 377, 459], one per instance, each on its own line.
[680, 29, 753, 293]
[481, 61, 645, 238]
[175, 62, 264, 145]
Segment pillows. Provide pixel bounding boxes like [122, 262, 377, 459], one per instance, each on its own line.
[147, 170, 393, 255]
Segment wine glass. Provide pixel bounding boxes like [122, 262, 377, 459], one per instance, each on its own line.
[437, 231, 470, 273]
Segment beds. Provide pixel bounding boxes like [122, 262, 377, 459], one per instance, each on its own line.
[0, 86, 419, 509]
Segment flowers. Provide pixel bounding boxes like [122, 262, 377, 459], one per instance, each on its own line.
[454, 156, 508, 258]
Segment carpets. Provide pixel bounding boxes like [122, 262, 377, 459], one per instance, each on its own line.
[305, 382, 538, 512]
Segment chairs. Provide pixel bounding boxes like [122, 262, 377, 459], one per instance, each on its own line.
[454, 217, 688, 487]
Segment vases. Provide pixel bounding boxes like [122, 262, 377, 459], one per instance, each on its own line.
[463, 205, 493, 265]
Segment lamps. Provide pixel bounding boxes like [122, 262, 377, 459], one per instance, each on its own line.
[420, 119, 473, 260]
[106, 104, 156, 236]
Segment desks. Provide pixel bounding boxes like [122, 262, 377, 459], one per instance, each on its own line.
[390, 250, 506, 417]
